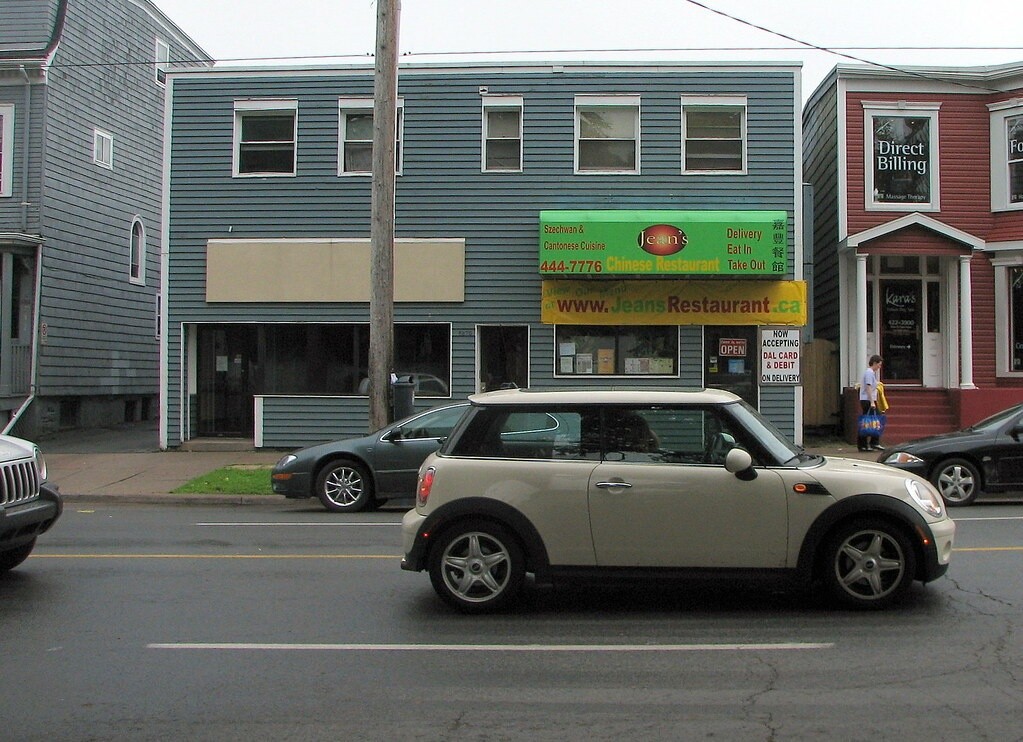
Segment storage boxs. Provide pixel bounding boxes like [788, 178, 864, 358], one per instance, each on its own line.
[597, 348, 615, 374]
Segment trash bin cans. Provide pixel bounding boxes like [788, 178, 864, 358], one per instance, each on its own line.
[390, 376, 418, 422]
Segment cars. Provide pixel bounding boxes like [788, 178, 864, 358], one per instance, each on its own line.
[272, 399, 585, 512]
[877, 400, 1023, 507]
[400, 385, 956, 614]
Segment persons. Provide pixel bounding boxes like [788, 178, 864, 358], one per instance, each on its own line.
[614, 411, 661, 452]
[857, 354, 886, 452]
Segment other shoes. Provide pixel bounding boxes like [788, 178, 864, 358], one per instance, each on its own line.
[870, 444, 885, 450]
[859, 447, 873, 451]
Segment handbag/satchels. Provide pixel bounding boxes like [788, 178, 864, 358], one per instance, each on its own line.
[857, 407, 885, 437]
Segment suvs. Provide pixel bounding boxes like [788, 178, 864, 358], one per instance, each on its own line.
[0, 433, 63, 579]
